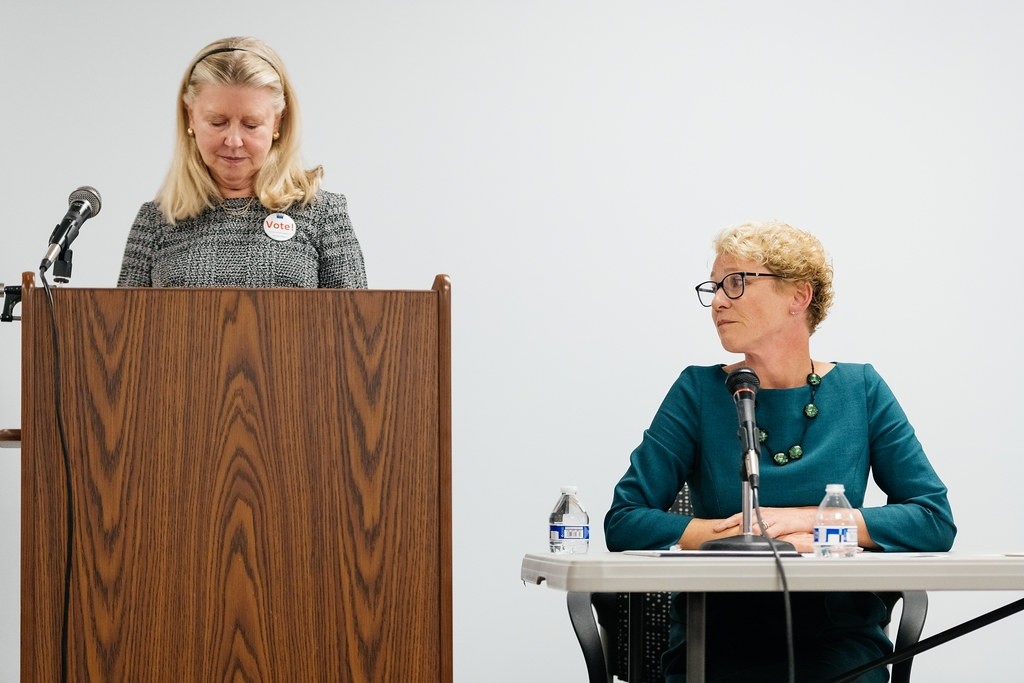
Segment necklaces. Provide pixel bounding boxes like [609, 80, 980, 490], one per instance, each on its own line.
[737, 357, 822, 464]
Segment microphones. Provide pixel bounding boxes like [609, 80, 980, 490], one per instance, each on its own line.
[725, 366, 759, 490]
[39, 185, 101, 272]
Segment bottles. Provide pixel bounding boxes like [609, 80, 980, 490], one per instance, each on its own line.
[813, 483, 858, 561]
[548, 487, 591, 557]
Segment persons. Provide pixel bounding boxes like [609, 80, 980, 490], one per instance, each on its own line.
[600, 221, 957, 683]
[117, 35, 369, 290]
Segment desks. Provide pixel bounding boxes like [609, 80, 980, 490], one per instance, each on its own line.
[520, 550, 1024, 683]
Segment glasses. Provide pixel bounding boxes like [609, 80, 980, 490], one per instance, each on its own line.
[695, 272, 784, 307]
